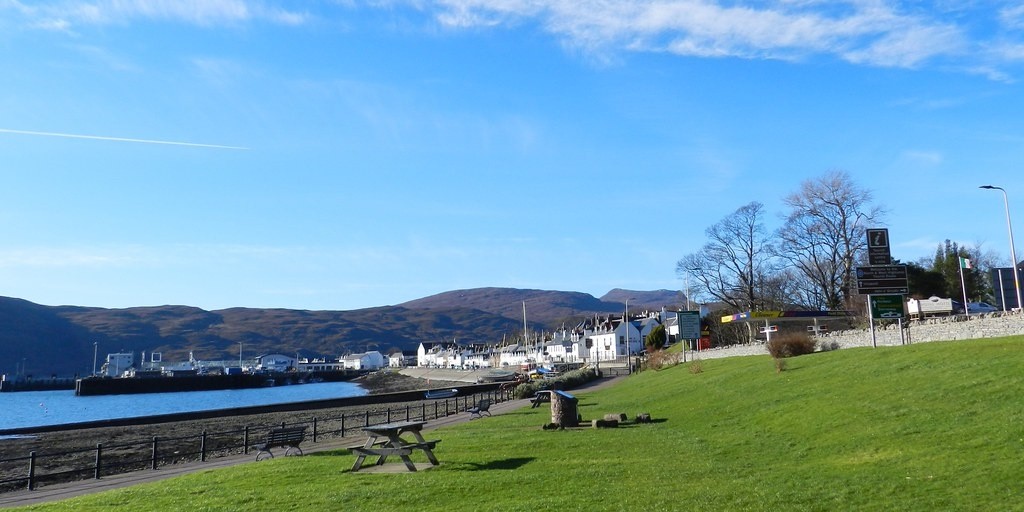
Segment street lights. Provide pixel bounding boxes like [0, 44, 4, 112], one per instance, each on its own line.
[625, 297, 637, 374]
[686, 267, 702, 353]
[979, 186, 1023, 311]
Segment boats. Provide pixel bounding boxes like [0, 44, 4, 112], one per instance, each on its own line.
[479, 370, 517, 382]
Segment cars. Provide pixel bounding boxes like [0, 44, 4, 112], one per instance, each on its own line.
[967, 302, 998, 312]
[936, 297, 965, 316]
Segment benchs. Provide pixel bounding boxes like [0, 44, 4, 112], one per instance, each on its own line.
[252, 424, 310, 460]
[467, 398, 492, 421]
[401, 438, 442, 450]
[347, 439, 388, 450]
[530, 399, 536, 403]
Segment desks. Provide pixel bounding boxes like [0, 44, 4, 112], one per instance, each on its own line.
[537, 391, 551, 408]
[360, 421, 427, 472]
